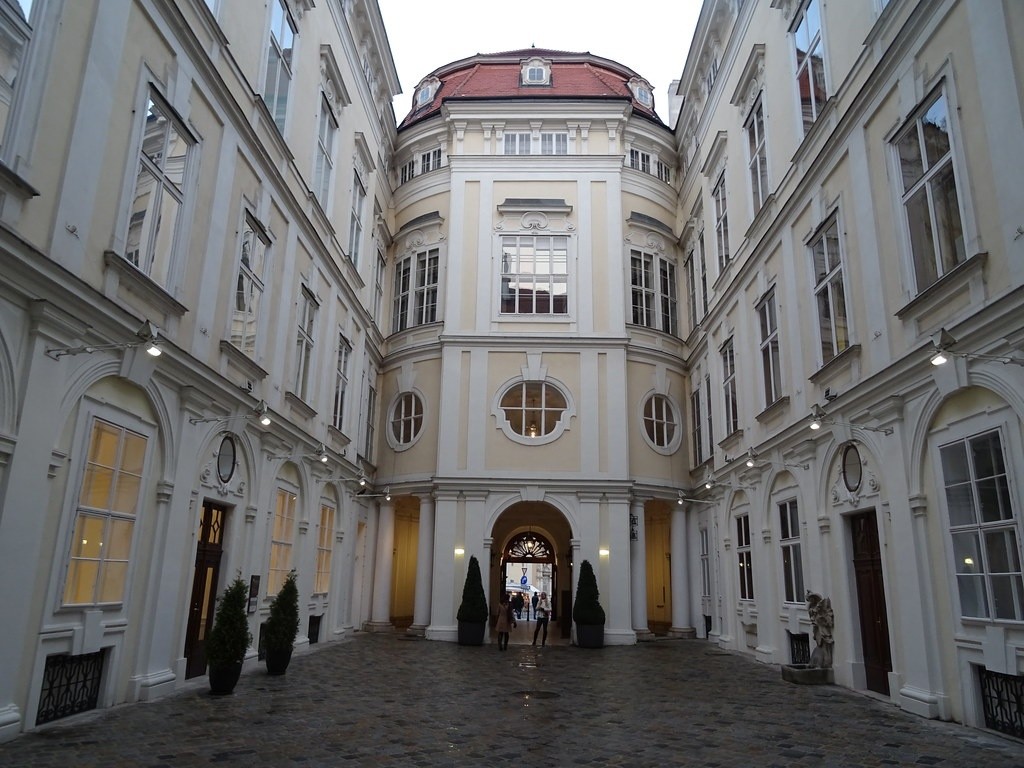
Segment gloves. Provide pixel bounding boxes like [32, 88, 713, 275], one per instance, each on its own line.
[514, 622, 517, 628]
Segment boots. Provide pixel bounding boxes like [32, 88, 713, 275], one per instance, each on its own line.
[504, 636, 509, 650]
[542, 632, 548, 647]
[498, 635, 503, 650]
[532, 631, 538, 645]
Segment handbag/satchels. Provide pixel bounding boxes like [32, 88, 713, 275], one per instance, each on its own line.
[507, 606, 513, 622]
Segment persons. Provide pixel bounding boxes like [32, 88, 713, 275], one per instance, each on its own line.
[510, 593, 530, 619]
[495, 594, 517, 651]
[532, 592, 539, 619]
[532, 592, 552, 646]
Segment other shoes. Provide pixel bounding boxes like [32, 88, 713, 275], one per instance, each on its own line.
[518, 617, 521, 619]
[534, 617, 536, 619]
